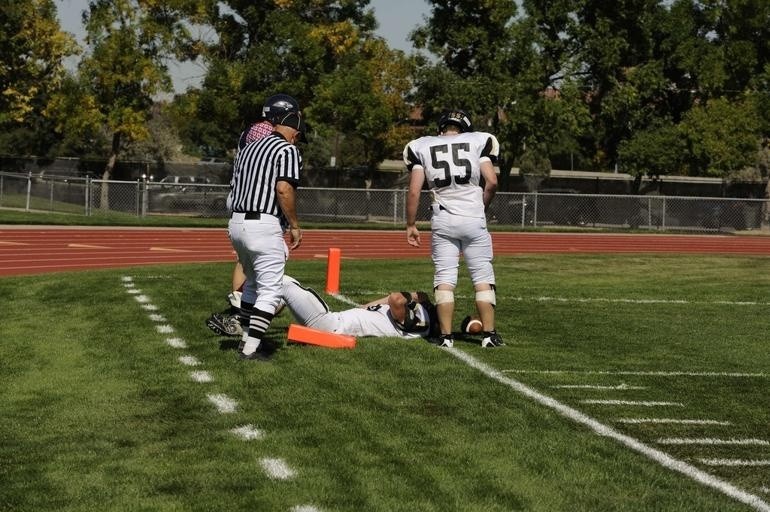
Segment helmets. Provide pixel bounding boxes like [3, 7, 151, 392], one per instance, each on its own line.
[262, 94, 303, 130]
[437, 108, 472, 131]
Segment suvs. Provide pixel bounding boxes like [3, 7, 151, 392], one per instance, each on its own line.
[147, 173, 221, 191]
[493, 188, 593, 225]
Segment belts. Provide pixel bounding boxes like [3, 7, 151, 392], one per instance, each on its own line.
[230, 211, 260, 219]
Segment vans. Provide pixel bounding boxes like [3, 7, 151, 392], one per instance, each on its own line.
[37, 170, 103, 197]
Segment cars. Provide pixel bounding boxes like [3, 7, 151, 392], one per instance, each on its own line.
[416, 196, 493, 221]
[140, 183, 232, 211]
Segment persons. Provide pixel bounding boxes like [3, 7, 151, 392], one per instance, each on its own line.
[232, 96, 299, 292]
[206, 275, 433, 340]
[402, 110, 500, 349]
[227, 112, 302, 361]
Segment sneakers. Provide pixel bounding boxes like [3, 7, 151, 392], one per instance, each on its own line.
[439, 335, 455, 348]
[206, 291, 271, 362]
[481, 330, 506, 347]
